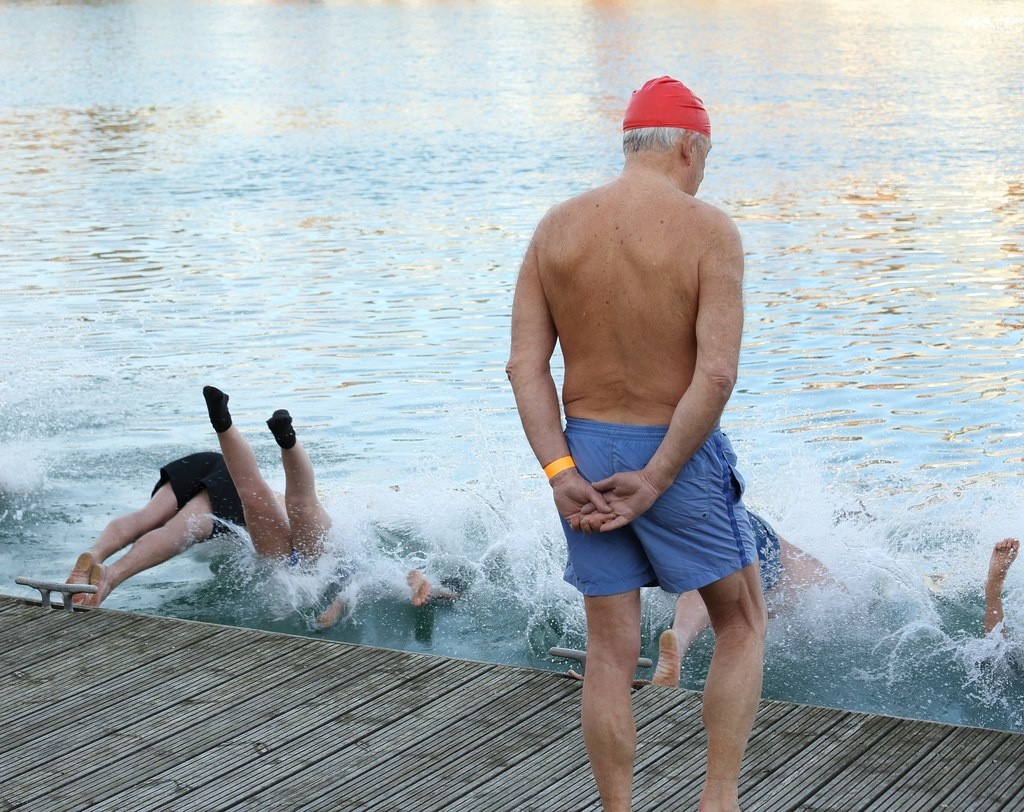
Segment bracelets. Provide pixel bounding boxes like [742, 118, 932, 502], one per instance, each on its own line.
[544, 456, 575, 480]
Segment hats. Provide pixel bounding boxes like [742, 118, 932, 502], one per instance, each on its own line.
[623, 75, 711, 137]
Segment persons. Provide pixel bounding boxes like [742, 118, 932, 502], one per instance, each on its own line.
[984, 539, 1020, 634]
[505, 75, 768, 812]
[203, 386, 333, 566]
[319, 569, 459, 630]
[651, 509, 846, 689]
[63, 451, 248, 607]
